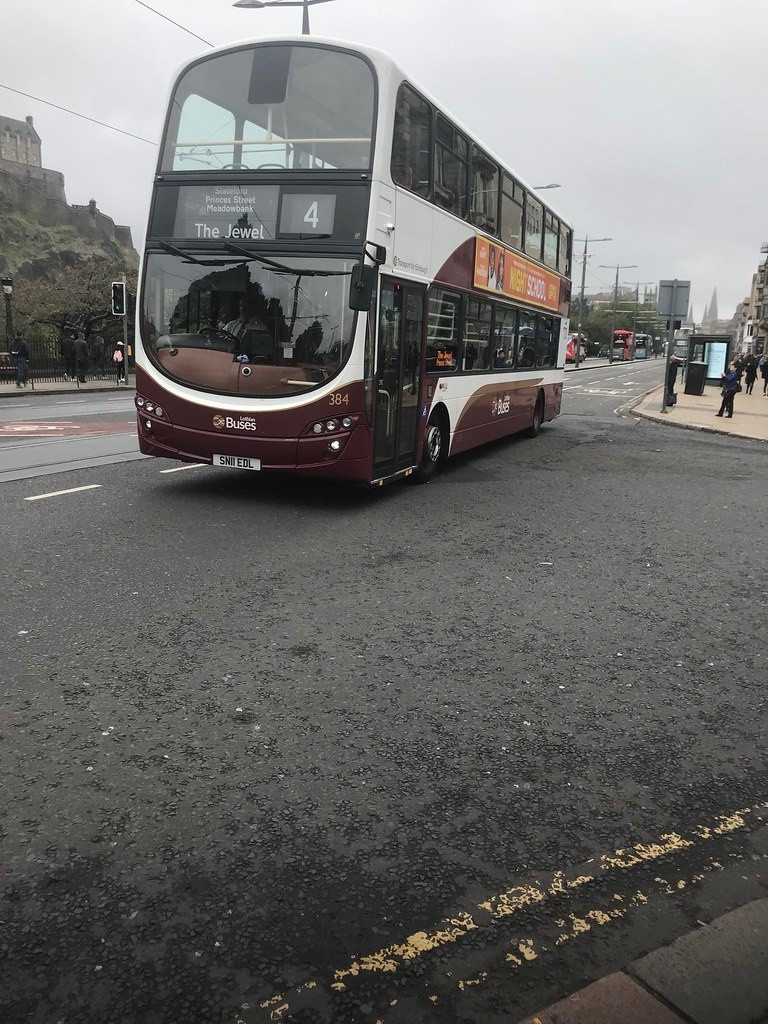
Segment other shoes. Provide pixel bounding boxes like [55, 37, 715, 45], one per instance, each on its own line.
[81, 381, 87, 383]
[17, 386, 22, 388]
[71, 377, 77, 381]
[725, 415, 731, 418]
[121, 378, 125, 381]
[667, 404, 673, 406]
[64, 373, 67, 380]
[715, 414, 723, 417]
[118, 380, 121, 382]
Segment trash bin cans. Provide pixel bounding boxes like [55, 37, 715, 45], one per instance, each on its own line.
[684, 359, 708, 395]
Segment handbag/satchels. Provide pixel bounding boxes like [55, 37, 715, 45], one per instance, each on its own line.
[113, 348, 123, 363]
[736, 384, 742, 392]
[666, 393, 678, 404]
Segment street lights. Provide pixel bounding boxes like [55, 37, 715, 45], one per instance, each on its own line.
[232, 0, 338, 37]
[1, 276, 16, 352]
[621, 281, 654, 361]
[598, 264, 637, 363]
[573, 235, 612, 368]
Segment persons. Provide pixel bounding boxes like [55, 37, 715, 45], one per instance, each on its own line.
[409, 340, 419, 395]
[725, 353, 768, 396]
[665, 356, 677, 406]
[392, 119, 416, 189]
[9, 331, 29, 388]
[72, 332, 88, 383]
[716, 364, 738, 418]
[488, 249, 505, 291]
[91, 343, 105, 376]
[60, 331, 77, 382]
[110, 341, 125, 383]
[494, 350, 506, 368]
[221, 299, 269, 341]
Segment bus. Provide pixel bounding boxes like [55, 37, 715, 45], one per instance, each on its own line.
[672, 327, 698, 366]
[609, 330, 654, 360]
[133, 37, 574, 488]
[566, 332, 587, 363]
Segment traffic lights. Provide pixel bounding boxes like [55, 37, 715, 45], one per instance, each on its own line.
[111, 281, 125, 316]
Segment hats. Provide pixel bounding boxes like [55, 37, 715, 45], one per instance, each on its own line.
[17, 332, 23, 336]
[78, 332, 85, 338]
[117, 341, 124, 345]
